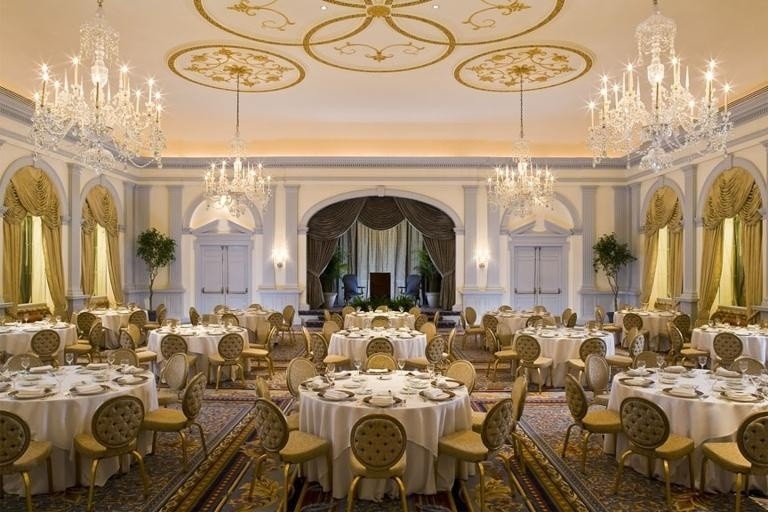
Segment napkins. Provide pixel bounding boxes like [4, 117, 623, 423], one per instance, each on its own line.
[369, 395, 393, 405]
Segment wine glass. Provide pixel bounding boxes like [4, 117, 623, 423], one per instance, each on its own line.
[1, 305, 269, 400]
[326, 303, 436, 388]
[489, 305, 768, 405]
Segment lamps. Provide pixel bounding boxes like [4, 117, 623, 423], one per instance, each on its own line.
[486, 72, 556, 215]
[590, 1, 735, 175]
[203, 72, 275, 220]
[29, 0, 168, 168]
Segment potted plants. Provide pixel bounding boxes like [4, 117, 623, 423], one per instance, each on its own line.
[591, 230, 637, 323]
[320, 247, 350, 309]
[135, 228, 177, 323]
[414, 249, 440, 309]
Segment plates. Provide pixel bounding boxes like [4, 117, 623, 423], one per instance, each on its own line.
[420, 389, 455, 401]
[301, 380, 336, 390]
[318, 390, 354, 400]
[365, 395, 402, 407]
[431, 379, 464, 390]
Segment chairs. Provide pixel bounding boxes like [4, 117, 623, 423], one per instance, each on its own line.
[142, 372, 208, 472]
[1, 304, 295, 405]
[342, 275, 364, 306]
[701, 413, 767, 510]
[74, 396, 148, 510]
[435, 400, 515, 511]
[471, 376, 528, 476]
[247, 400, 334, 511]
[560, 375, 625, 473]
[398, 275, 422, 306]
[612, 398, 694, 510]
[286, 309, 519, 399]
[348, 413, 407, 511]
[1, 410, 56, 511]
[498, 306, 767, 406]
